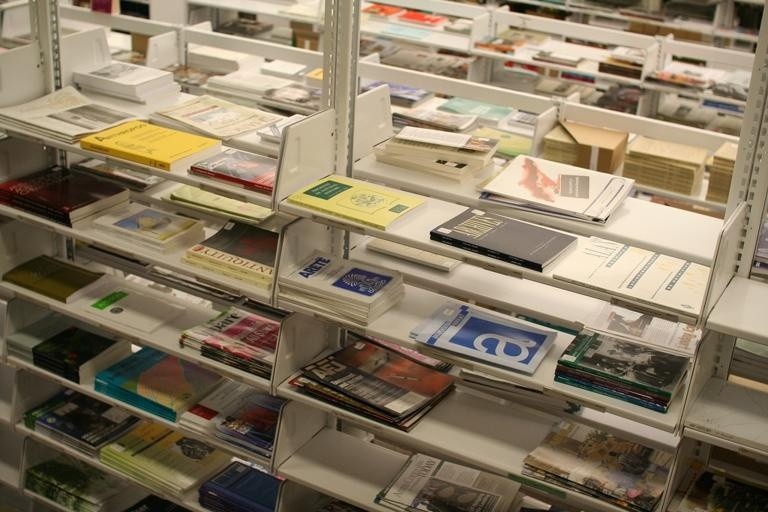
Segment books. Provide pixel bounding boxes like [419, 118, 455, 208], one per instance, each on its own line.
[1, 0, 768, 512]
